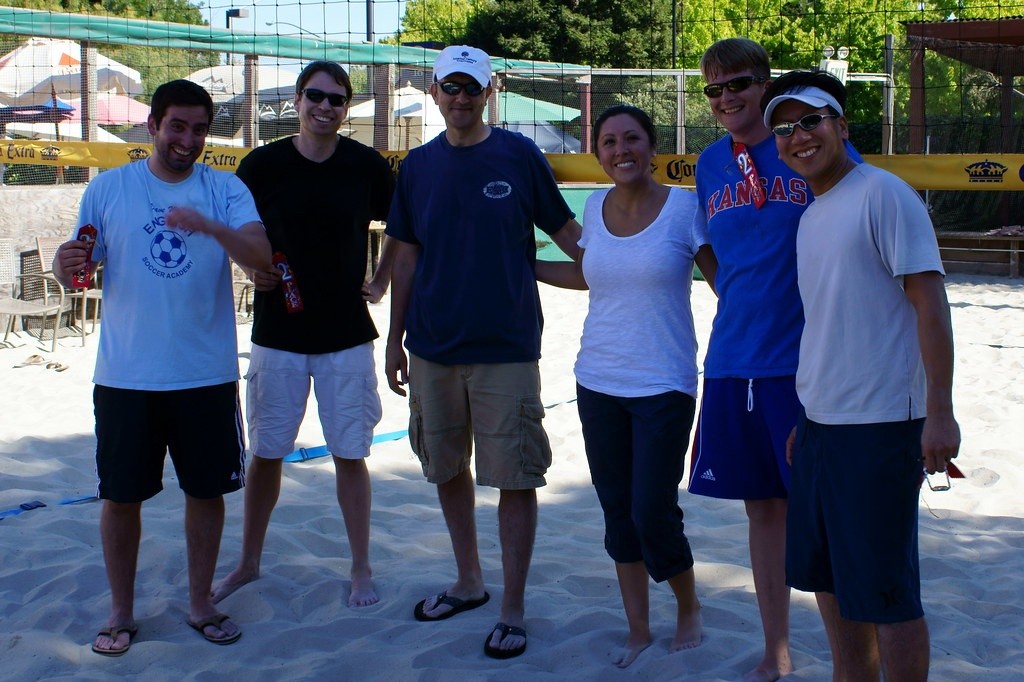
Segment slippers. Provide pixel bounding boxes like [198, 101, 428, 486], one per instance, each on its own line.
[92, 622, 137, 655]
[186, 612, 240, 645]
[414, 588, 490, 623]
[484, 623, 527, 660]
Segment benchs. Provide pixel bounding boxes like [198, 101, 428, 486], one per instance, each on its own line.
[934, 231, 1024, 279]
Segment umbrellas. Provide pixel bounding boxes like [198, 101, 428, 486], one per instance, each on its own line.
[0, 36, 582, 185]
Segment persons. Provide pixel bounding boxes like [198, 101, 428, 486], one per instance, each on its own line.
[759, 70, 961, 682]
[383, 45, 583, 659]
[208, 59, 395, 608]
[53, 78, 273, 657]
[535, 104, 717, 668]
[687, 37, 863, 682]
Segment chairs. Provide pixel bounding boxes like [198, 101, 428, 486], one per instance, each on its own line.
[0, 236, 105, 354]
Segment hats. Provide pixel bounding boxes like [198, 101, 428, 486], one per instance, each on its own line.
[432, 45, 493, 89]
[763, 85, 843, 130]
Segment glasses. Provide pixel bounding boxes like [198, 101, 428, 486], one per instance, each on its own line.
[703, 76, 767, 98]
[299, 88, 348, 107]
[772, 114, 837, 137]
[435, 80, 490, 97]
